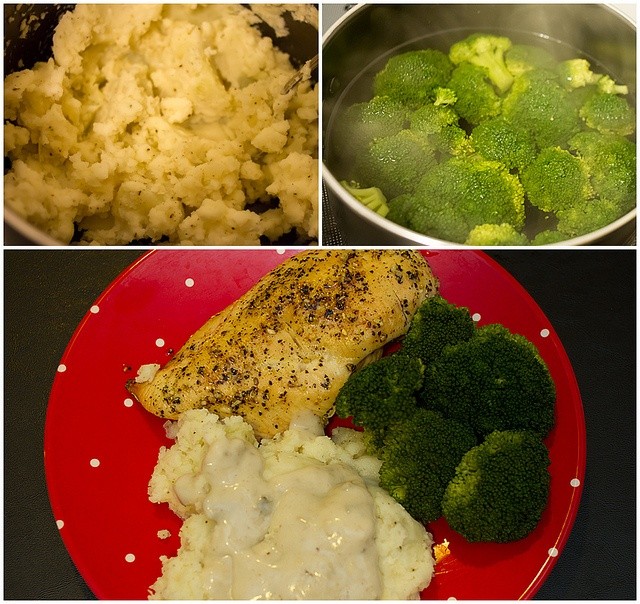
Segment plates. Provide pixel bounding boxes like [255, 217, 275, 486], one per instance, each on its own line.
[45, 249, 587, 599]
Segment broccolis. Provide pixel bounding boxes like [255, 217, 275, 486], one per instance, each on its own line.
[333, 294, 556, 543]
[330, 31, 635, 245]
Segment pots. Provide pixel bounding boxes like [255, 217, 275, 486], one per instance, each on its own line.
[320, 4, 636, 246]
